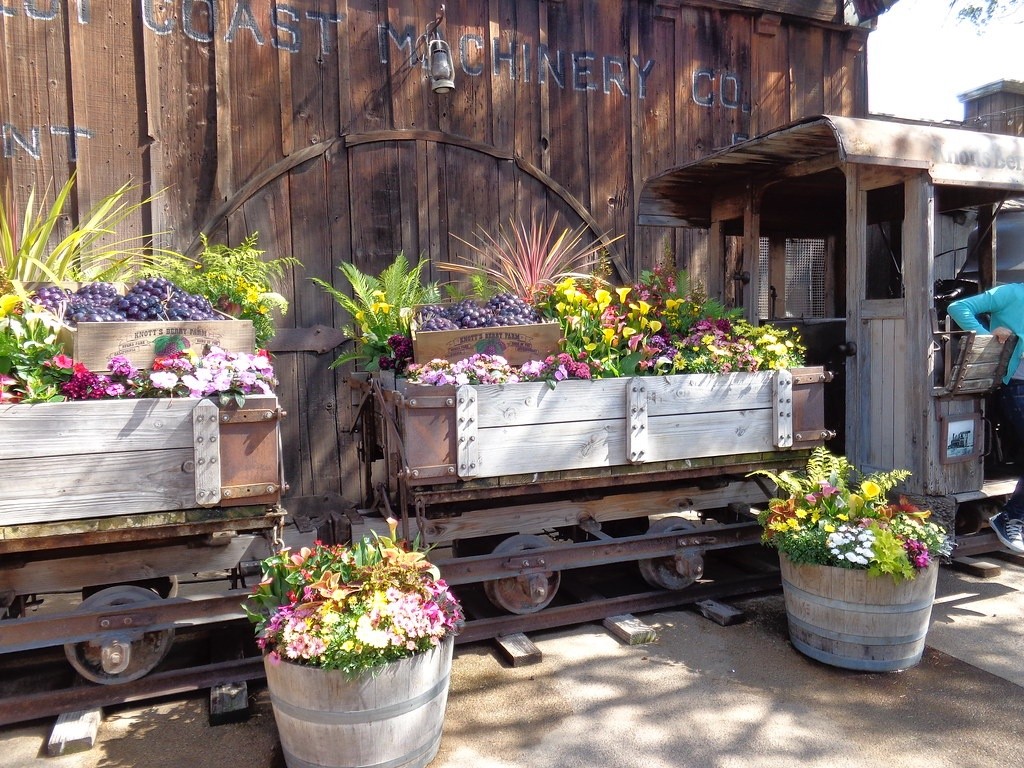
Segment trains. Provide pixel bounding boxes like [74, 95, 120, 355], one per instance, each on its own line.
[0, 112, 1024, 688]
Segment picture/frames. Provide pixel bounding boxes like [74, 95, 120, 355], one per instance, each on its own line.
[939, 414, 980, 464]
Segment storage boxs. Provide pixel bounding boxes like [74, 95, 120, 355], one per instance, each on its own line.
[409, 298, 561, 366]
[56, 309, 257, 381]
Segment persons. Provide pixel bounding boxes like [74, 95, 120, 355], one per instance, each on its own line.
[946, 281, 1024, 554]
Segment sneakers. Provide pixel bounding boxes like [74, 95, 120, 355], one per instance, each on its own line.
[988, 510, 1024, 553]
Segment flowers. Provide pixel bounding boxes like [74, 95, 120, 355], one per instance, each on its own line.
[308, 211, 806, 387]
[0, 172, 302, 404]
[744, 445, 956, 585]
[241, 517, 465, 683]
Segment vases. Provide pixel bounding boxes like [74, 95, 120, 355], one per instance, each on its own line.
[777, 550, 940, 671]
[261, 636, 454, 768]
